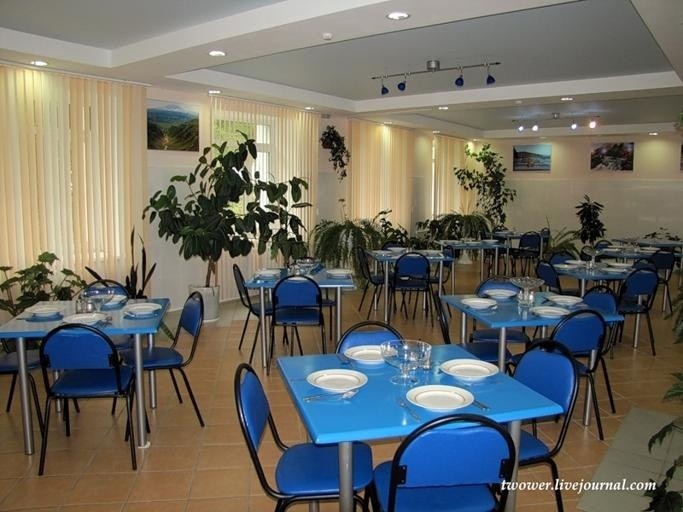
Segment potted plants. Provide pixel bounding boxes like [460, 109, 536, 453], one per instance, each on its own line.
[139, 128, 315, 327]
[432, 208, 490, 266]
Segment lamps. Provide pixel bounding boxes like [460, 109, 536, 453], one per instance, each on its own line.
[370, 61, 503, 96]
[511, 114, 602, 133]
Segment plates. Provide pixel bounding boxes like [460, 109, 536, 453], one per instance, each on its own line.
[125, 302, 163, 317]
[440, 357, 499, 383]
[344, 344, 396, 365]
[23, 304, 65, 318]
[91, 294, 128, 306]
[253, 230, 661, 318]
[306, 368, 369, 395]
[63, 313, 107, 326]
[406, 383, 474, 413]
